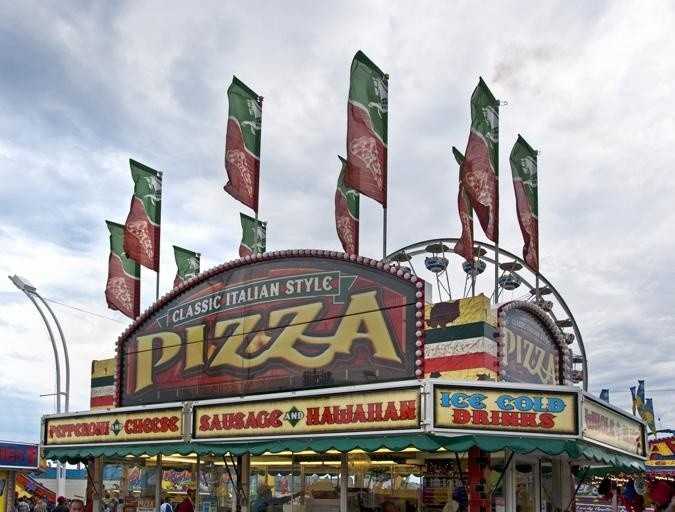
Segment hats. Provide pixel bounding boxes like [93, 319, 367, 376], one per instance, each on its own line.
[257, 484, 274, 495]
[188, 482, 197, 490]
[57, 496, 65, 502]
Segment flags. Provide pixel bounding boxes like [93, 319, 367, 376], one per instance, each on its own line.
[453, 76, 500, 267]
[630, 380, 658, 439]
[335, 50, 389, 255]
[239, 212, 267, 257]
[105, 159, 163, 320]
[509, 133, 538, 271]
[600, 388, 609, 403]
[173, 245, 201, 287]
[224, 75, 263, 213]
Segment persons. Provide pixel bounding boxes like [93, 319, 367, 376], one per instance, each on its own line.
[160, 479, 197, 512]
[14, 483, 118, 512]
[621, 475, 635, 512]
[252, 485, 307, 512]
